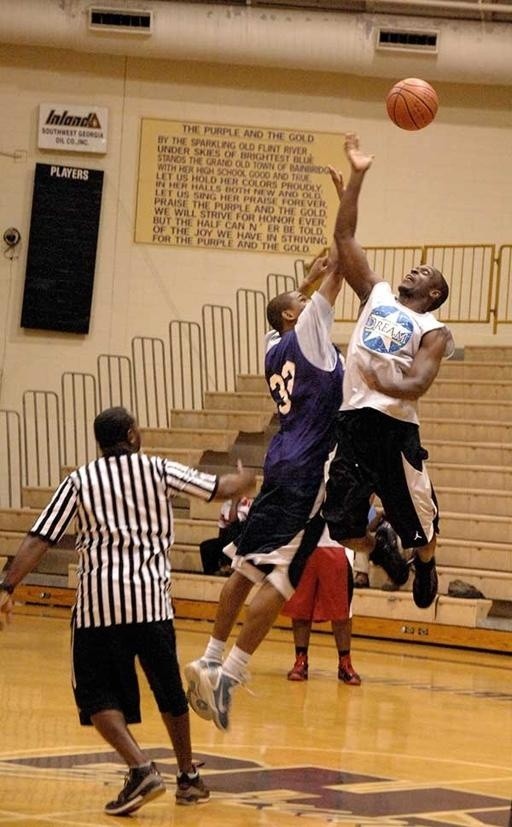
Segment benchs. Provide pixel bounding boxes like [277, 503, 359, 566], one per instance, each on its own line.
[0, 336, 511, 656]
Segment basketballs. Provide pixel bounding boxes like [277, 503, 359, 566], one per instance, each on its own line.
[386, 78, 437, 129]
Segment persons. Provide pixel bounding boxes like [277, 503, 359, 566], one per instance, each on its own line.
[355, 493, 408, 589]
[197, 475, 256, 576]
[0, 402, 257, 815]
[185, 163, 349, 736]
[281, 441, 366, 687]
[327, 125, 457, 613]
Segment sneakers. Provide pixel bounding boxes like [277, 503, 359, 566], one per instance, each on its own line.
[199, 665, 257, 732]
[185, 657, 220, 721]
[104, 761, 168, 815]
[287, 655, 309, 681]
[367, 527, 410, 587]
[338, 653, 361, 687]
[407, 548, 439, 609]
[175, 763, 210, 806]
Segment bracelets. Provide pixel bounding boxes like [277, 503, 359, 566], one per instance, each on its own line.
[0, 582, 17, 594]
[303, 278, 313, 289]
